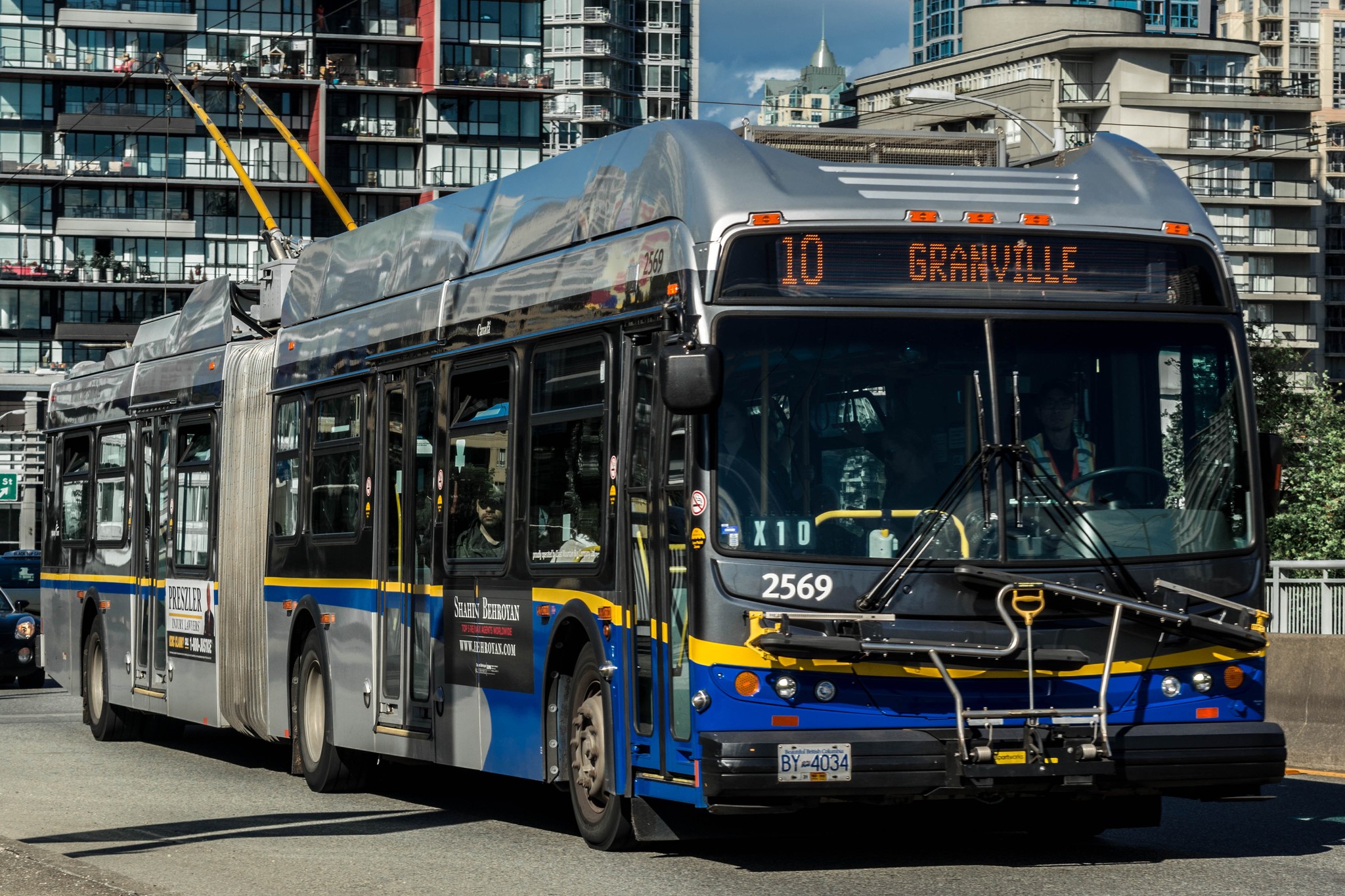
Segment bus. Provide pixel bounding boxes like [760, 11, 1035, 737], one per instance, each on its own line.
[42, 50, 1290, 853]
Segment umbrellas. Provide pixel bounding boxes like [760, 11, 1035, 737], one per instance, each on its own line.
[23, 234, 28, 279]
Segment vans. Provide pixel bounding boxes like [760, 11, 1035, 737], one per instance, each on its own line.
[0, 549, 41, 613]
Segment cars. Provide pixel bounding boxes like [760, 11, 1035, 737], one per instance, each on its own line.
[0, 586, 45, 689]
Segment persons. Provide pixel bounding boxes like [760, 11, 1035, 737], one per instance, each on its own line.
[349, 115, 356, 135]
[846, 497, 882, 540]
[1022, 381, 1119, 554]
[757, 412, 815, 516]
[317, 3, 325, 27]
[456, 481, 504, 558]
[721, 389, 791, 536]
[283, 63, 292, 71]
[865, 423, 951, 558]
[787, 484, 866, 557]
